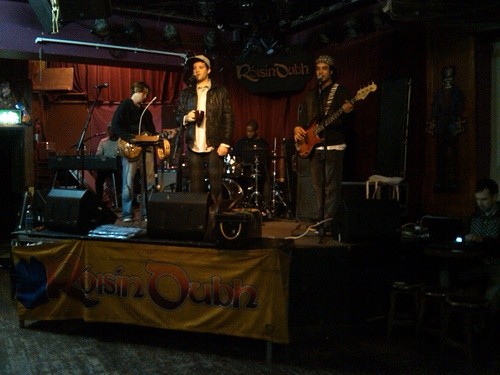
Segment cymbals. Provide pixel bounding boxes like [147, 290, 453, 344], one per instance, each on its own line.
[243, 148, 265, 151]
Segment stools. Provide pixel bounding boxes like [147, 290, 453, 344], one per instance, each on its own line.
[389, 282, 487, 375]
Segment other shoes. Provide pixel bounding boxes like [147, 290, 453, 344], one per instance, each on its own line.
[309, 226, 318, 232]
[315, 228, 331, 235]
[123, 218, 132, 222]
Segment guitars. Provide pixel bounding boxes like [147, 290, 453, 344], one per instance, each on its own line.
[119, 130, 172, 160]
[294, 80, 377, 159]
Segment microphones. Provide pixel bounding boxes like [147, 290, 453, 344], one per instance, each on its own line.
[93, 82, 108, 88]
[186, 76, 196, 84]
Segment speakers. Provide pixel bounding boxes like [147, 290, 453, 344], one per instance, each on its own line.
[330, 197, 402, 242]
[43, 188, 117, 235]
[421, 215, 463, 242]
[147, 188, 211, 239]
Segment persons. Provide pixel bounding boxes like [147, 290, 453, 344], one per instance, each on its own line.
[93, 125, 124, 211]
[178, 54, 232, 207]
[110, 81, 176, 223]
[229, 120, 271, 209]
[290, 54, 354, 237]
[462, 177, 500, 305]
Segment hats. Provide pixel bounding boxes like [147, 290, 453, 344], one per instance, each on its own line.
[186, 55, 210, 69]
[316, 56, 335, 68]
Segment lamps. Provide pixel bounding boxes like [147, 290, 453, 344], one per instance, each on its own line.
[93, 13, 111, 38]
[342, 13, 365, 39]
[124, 13, 146, 44]
[371, 4, 385, 27]
[163, 23, 183, 47]
[316, 21, 336, 45]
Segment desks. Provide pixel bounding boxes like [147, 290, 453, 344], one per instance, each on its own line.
[394, 241, 484, 327]
[10, 230, 299, 363]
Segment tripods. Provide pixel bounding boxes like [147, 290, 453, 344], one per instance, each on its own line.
[245, 148, 295, 220]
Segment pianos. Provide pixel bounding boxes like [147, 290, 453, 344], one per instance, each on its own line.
[47, 156, 122, 209]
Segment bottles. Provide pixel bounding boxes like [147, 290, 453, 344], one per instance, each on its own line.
[25, 205, 34, 234]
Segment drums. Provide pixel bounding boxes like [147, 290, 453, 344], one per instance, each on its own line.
[202, 178, 244, 201]
[243, 158, 266, 176]
